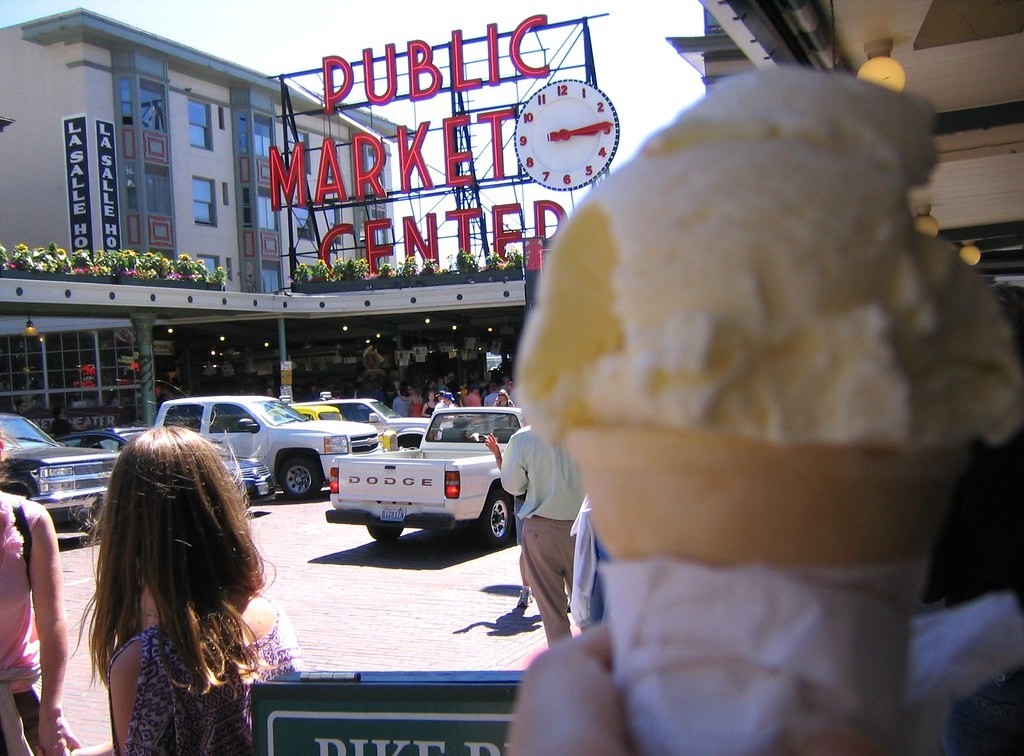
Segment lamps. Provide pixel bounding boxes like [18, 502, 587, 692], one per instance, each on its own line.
[22, 316, 39, 336]
[913, 203, 939, 237]
[856, 39, 906, 93]
[951, 241, 980, 267]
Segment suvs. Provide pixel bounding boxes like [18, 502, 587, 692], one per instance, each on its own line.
[0, 412, 121, 524]
[293, 391, 450, 452]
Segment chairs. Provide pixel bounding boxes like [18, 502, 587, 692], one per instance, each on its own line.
[442, 428, 466, 441]
[493, 429, 514, 443]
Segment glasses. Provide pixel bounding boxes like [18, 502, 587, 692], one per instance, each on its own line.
[498, 394, 505, 396]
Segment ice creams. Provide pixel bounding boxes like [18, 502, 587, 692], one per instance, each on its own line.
[506, 63, 1023, 572]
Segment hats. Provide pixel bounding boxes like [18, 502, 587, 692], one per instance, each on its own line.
[444, 392, 455, 401]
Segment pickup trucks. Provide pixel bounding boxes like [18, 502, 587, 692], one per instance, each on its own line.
[325, 406, 524, 547]
[154, 394, 383, 499]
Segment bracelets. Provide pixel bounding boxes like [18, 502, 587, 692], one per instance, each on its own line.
[496, 456, 501, 459]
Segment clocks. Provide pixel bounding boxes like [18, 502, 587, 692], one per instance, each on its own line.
[513, 78, 620, 192]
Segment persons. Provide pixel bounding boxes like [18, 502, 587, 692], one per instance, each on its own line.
[293, 383, 325, 402]
[0, 425, 303, 756]
[508, 624, 884, 756]
[484, 409, 586, 646]
[14, 399, 27, 416]
[392, 373, 513, 418]
[264, 384, 278, 398]
[52, 408, 87, 436]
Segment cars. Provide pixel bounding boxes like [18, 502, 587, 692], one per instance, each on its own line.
[289, 406, 399, 453]
[53, 426, 276, 509]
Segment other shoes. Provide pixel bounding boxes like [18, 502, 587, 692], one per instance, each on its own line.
[518, 590, 529, 606]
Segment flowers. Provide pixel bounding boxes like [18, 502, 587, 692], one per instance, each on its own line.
[291, 247, 526, 285]
[0, 240, 227, 287]
